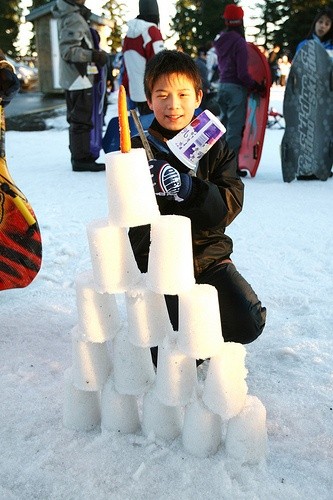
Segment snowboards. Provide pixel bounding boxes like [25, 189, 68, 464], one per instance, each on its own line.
[280, 42, 333, 182]
[238, 42, 271, 178]
[90, 30, 108, 159]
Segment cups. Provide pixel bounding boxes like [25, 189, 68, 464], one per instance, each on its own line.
[165, 109, 227, 170]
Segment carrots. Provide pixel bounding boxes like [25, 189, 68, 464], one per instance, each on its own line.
[118, 85, 131, 153]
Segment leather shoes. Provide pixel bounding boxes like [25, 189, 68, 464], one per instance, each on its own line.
[71, 159, 105, 172]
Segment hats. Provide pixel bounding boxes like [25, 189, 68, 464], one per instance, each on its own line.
[221, 4, 244, 19]
[139, 0, 159, 16]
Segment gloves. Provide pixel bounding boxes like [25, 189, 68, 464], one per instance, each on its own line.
[148, 159, 192, 204]
